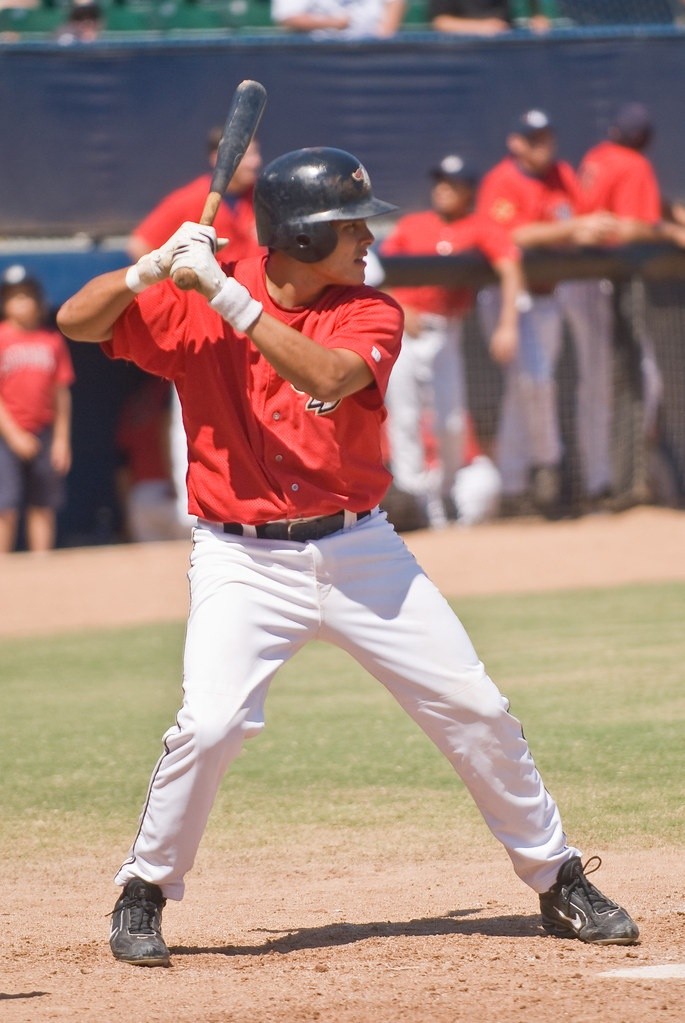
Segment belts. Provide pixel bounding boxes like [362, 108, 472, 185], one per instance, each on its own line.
[223, 510, 370, 543]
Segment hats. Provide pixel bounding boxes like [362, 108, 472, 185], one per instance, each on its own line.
[433, 151, 477, 178]
[509, 108, 551, 135]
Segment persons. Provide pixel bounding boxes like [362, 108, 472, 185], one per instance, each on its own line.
[56, 0, 680, 43]
[480, 102, 684, 521]
[118, 120, 267, 529]
[377, 151, 521, 528]
[0, 268, 75, 554]
[56, 146, 640, 967]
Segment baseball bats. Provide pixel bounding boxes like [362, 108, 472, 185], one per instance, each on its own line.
[172, 79, 268, 291]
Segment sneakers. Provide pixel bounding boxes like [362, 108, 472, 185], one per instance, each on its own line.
[540, 855, 640, 944]
[104, 877, 175, 967]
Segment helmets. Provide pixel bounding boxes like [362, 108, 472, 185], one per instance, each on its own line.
[252, 146, 398, 263]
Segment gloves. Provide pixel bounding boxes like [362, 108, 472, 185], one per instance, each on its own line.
[170, 242, 262, 332]
[125, 220, 229, 294]
